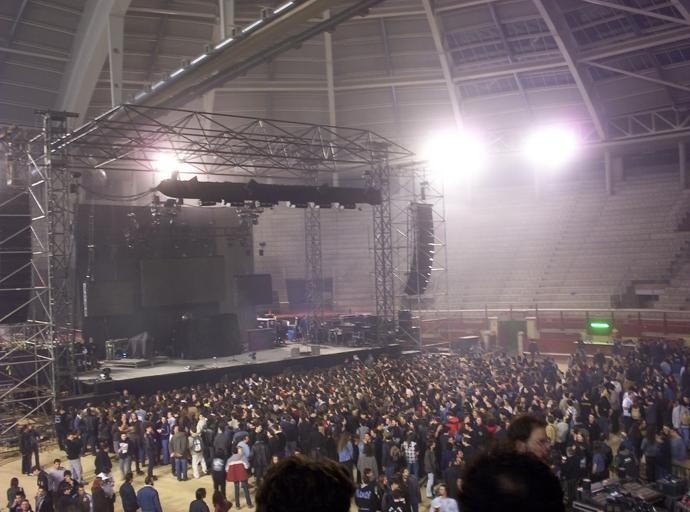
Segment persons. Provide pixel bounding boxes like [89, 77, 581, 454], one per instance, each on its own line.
[4, 330, 689, 512]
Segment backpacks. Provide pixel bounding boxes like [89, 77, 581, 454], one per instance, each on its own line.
[190, 435, 202, 453]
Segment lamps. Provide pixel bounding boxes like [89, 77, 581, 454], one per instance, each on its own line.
[100, 367, 114, 381]
[159, 175, 382, 213]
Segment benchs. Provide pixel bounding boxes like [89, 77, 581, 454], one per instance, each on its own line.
[255, 184, 690, 320]
